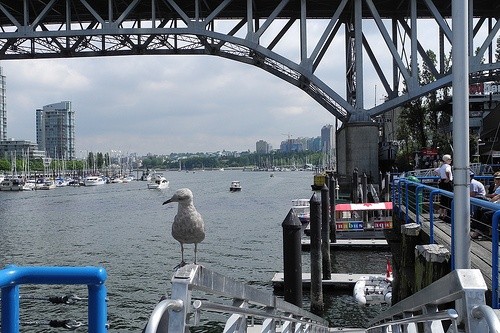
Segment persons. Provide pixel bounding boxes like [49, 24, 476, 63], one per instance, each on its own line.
[423, 155, 443, 167]
[434, 154, 453, 221]
[469, 170, 500, 239]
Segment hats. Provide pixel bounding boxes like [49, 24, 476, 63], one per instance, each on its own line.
[470, 171, 474, 176]
[494, 172, 500, 178]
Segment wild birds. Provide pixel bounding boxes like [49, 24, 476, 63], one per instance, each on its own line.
[162, 188, 206, 270]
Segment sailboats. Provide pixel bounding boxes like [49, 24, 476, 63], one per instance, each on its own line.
[0, 146, 135, 190]
[253, 155, 323, 172]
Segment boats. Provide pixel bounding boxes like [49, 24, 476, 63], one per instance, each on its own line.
[291, 199, 310, 222]
[230, 180, 241, 191]
[141, 167, 170, 189]
[310, 174, 328, 190]
[353, 274, 392, 304]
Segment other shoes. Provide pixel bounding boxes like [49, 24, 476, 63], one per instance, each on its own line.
[471, 229, 481, 237]
